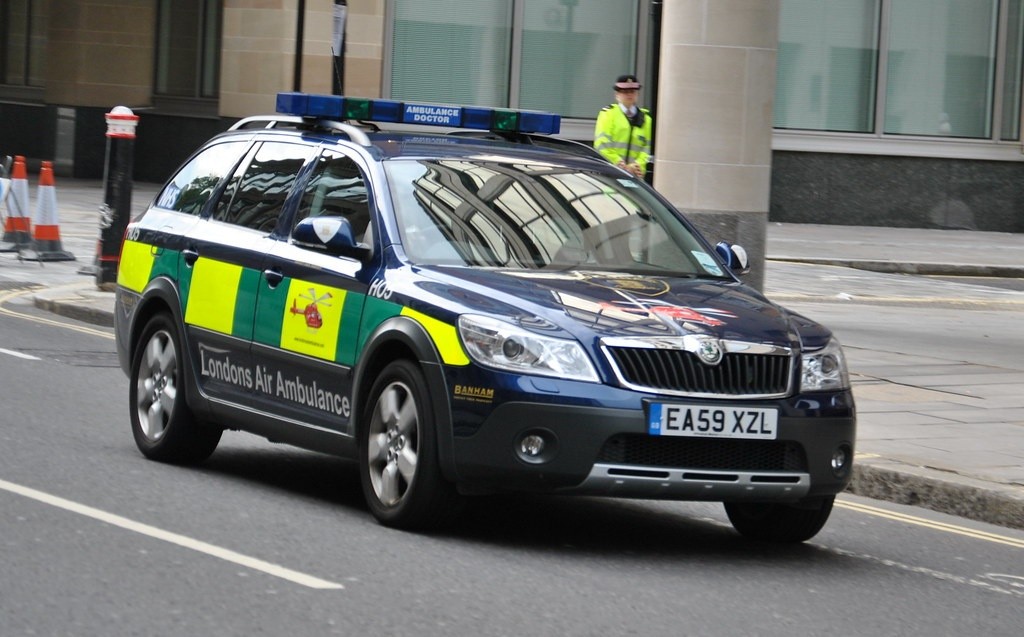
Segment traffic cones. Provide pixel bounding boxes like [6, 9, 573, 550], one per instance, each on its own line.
[18, 160, 76, 262]
[0, 156, 32, 251]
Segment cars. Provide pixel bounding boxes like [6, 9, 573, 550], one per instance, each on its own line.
[114, 92, 858, 543]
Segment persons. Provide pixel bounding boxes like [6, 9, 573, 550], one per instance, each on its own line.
[593, 75, 652, 244]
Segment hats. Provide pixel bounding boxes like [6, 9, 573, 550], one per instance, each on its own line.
[613, 75, 642, 92]
